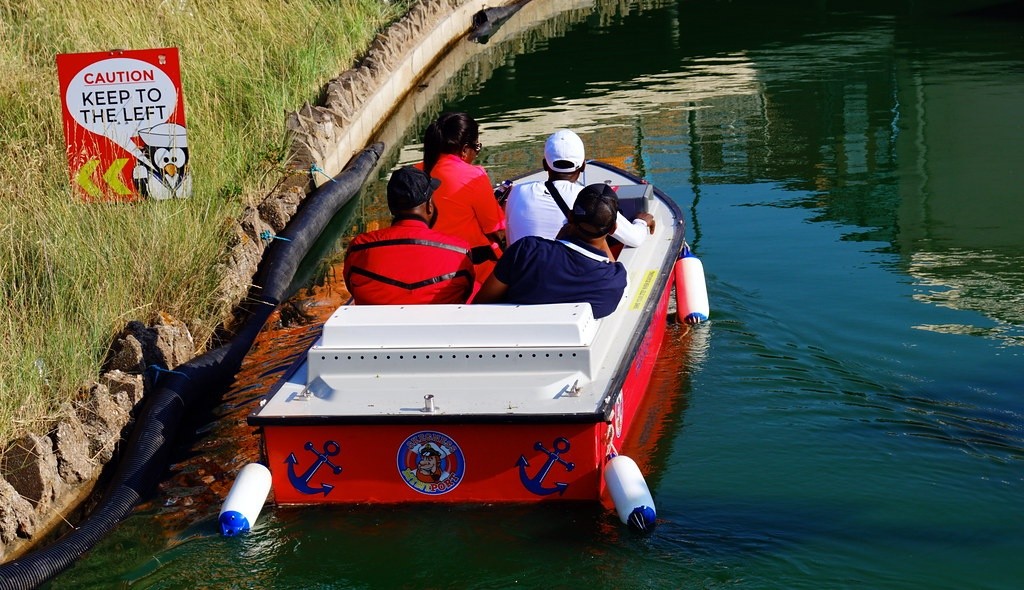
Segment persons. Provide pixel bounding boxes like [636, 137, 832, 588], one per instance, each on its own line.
[505, 129, 656, 248]
[343, 166, 476, 305]
[415, 112, 506, 284]
[471, 184, 627, 320]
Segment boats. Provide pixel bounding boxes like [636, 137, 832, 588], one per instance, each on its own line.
[218, 158, 710, 538]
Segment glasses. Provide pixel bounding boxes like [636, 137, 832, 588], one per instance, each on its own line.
[464, 140, 482, 154]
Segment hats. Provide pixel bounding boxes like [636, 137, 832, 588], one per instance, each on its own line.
[573, 183, 618, 237]
[387, 167, 440, 209]
[543, 130, 585, 173]
